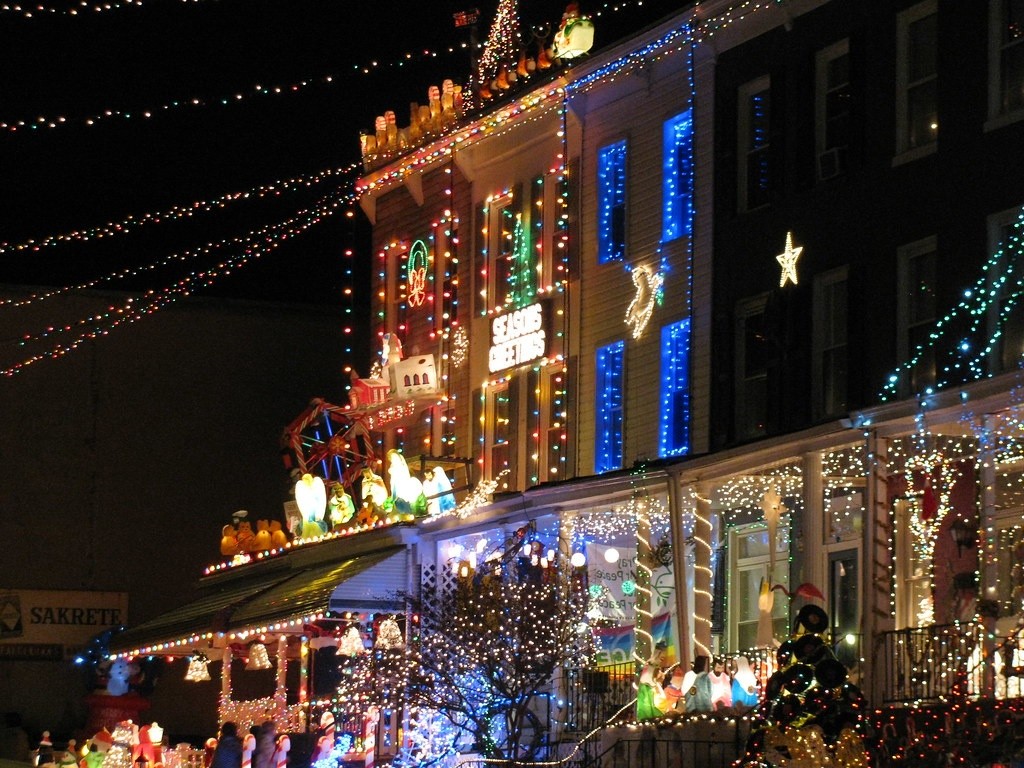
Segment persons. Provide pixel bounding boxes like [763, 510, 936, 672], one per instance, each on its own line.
[222, 519, 285, 554]
[296, 451, 456, 538]
[212, 721, 243, 768]
[249, 721, 278, 768]
[637, 643, 758, 722]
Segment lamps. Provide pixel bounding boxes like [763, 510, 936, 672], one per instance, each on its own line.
[374, 616, 404, 650]
[182, 657, 212, 683]
[333, 627, 365, 657]
[242, 641, 273, 671]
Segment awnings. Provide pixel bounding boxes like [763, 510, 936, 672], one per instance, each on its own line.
[100, 549, 403, 658]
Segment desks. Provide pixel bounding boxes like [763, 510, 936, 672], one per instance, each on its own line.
[405, 455, 475, 510]
